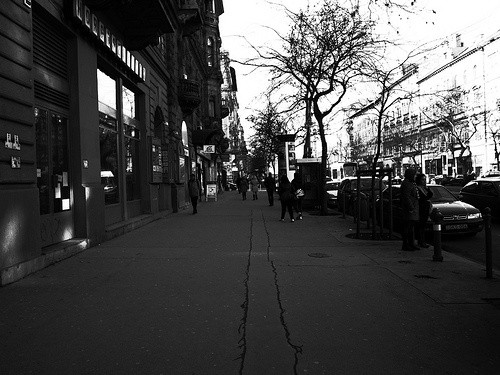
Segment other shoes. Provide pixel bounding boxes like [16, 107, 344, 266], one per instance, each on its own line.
[417, 241, 429, 248]
[291, 218, 295, 222]
[296, 215, 303, 220]
[408, 242, 420, 251]
[401, 243, 413, 252]
[424, 238, 431, 244]
[281, 216, 284, 222]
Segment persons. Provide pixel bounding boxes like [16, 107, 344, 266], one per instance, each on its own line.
[264, 173, 275, 206]
[279, 175, 294, 222]
[187, 174, 201, 214]
[237, 174, 259, 200]
[292, 173, 303, 220]
[415, 174, 433, 248]
[401, 169, 420, 251]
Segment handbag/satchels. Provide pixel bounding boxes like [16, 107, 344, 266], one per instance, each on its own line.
[294, 188, 305, 200]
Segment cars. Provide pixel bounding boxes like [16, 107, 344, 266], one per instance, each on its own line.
[376, 184, 484, 238]
[327, 181, 340, 205]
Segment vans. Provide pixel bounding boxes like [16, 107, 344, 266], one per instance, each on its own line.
[458, 177, 500, 218]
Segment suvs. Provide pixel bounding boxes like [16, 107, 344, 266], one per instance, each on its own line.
[336, 176, 387, 216]
